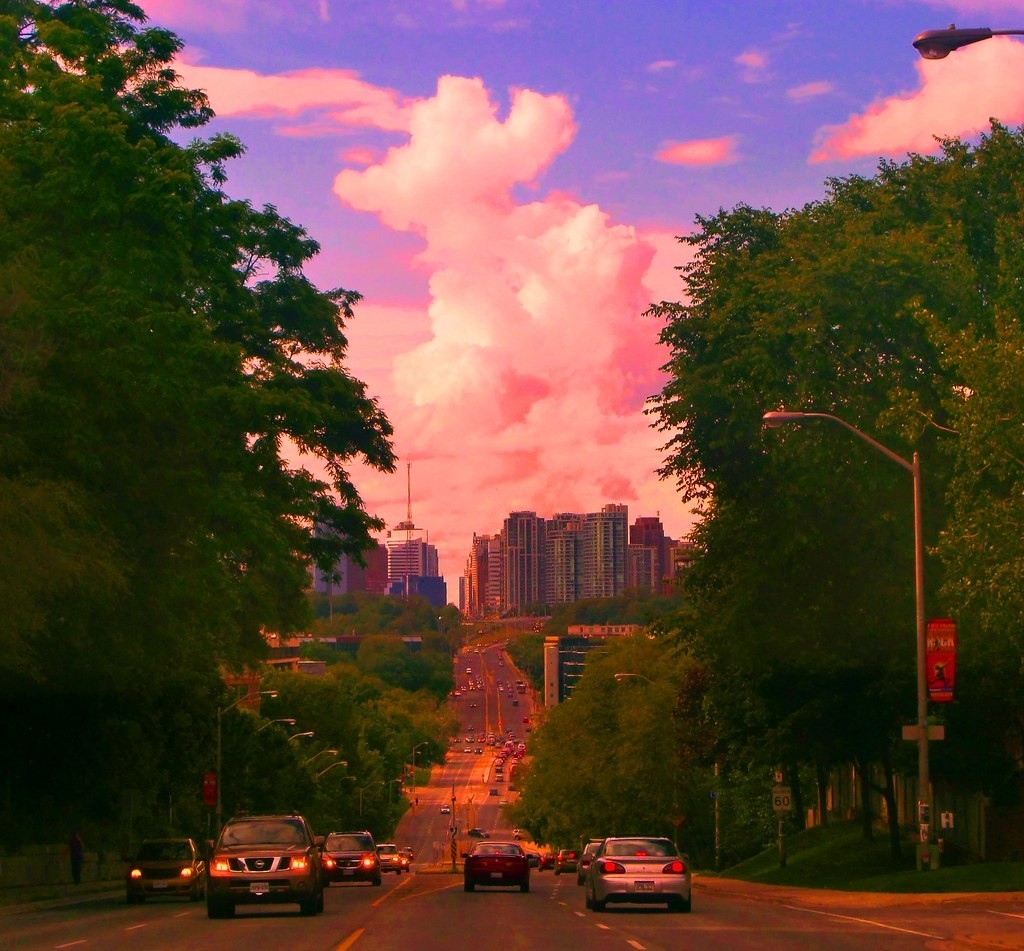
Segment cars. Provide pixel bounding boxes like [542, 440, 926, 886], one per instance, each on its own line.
[399, 846, 416, 873]
[581, 836, 692, 915]
[511, 829, 529, 840]
[469, 826, 490, 839]
[523, 849, 582, 876]
[440, 804, 450, 815]
[451, 636, 532, 808]
[461, 840, 533, 895]
[121, 836, 205, 904]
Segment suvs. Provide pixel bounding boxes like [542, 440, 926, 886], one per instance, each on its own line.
[203, 811, 326, 920]
[320, 830, 382, 887]
[376, 842, 401, 875]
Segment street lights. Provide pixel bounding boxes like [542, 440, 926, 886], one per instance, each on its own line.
[389, 778, 402, 815]
[404, 750, 422, 800]
[412, 741, 429, 800]
[314, 759, 349, 835]
[615, 673, 681, 849]
[244, 715, 297, 817]
[759, 408, 931, 872]
[214, 688, 280, 842]
[359, 780, 385, 817]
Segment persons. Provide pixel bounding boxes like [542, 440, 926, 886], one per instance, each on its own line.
[70, 831, 84, 885]
